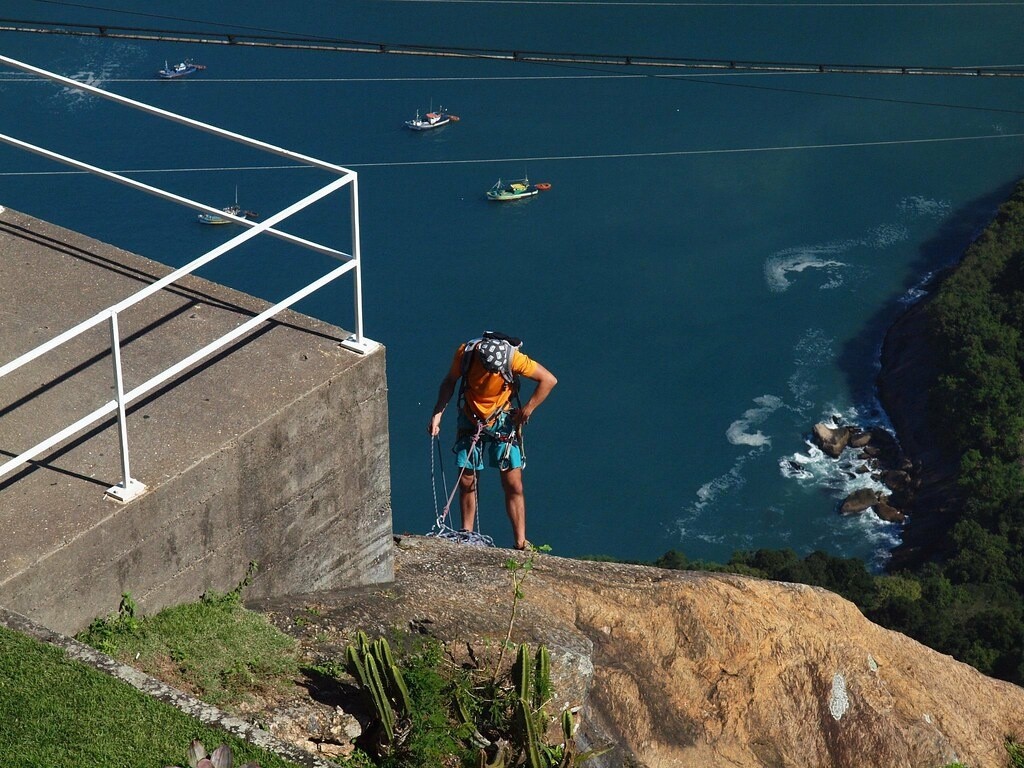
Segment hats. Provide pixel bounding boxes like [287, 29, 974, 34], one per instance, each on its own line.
[478, 339, 508, 373]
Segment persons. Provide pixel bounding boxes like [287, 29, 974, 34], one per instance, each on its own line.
[427, 330, 558, 551]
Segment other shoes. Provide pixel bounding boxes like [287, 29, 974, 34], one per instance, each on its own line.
[513, 540, 532, 552]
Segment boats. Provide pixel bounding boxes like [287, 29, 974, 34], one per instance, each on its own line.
[197, 186, 246, 224]
[487, 174, 539, 201]
[537, 183, 551, 190]
[405, 105, 450, 130]
[244, 211, 258, 217]
[159, 61, 196, 79]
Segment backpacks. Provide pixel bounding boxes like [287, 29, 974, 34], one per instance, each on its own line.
[461, 331, 523, 402]
